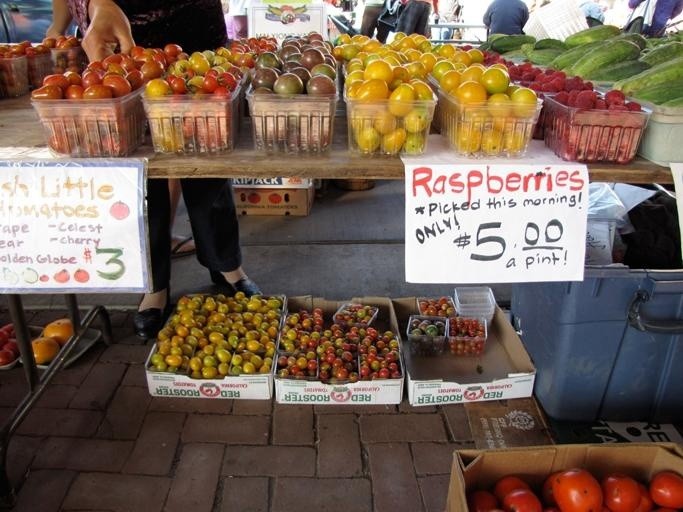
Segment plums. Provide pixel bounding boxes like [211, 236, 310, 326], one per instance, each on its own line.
[250, 31, 336, 97]
[0, 323, 20, 366]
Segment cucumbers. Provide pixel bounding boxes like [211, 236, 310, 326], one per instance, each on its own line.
[479, 16, 683, 108]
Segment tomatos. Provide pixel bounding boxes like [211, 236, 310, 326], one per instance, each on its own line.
[110, 202, 129, 220]
[150, 292, 288, 380]
[276, 302, 401, 380]
[75, 269, 89, 282]
[471, 469, 683, 512]
[333, 33, 537, 154]
[407, 296, 485, 356]
[0, 35, 278, 156]
[54, 270, 70, 283]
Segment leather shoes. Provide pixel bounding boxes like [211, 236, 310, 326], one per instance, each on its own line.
[134, 282, 172, 340]
[209, 263, 262, 297]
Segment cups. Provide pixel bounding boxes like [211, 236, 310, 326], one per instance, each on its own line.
[434, 15, 438, 24]
[0, 48, 84, 100]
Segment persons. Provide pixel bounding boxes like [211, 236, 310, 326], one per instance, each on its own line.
[482, 0, 528, 42]
[46, 0, 198, 259]
[623, 0, 683, 39]
[579, 2, 608, 29]
[221, 1, 246, 42]
[81, 0, 264, 343]
[432, 1, 463, 40]
[374, 0, 431, 43]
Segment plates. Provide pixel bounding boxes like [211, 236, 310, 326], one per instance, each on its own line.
[19, 327, 102, 372]
[0, 323, 44, 371]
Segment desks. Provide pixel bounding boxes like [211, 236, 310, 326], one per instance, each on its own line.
[0, 80, 683, 512]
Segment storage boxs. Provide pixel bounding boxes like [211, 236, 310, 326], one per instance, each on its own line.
[0, 40, 683, 169]
[507, 238, 683, 433]
[444, 439, 682, 512]
[141, 290, 538, 407]
[226, 173, 315, 218]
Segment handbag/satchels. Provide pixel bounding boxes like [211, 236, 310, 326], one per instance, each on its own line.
[620, 0, 657, 37]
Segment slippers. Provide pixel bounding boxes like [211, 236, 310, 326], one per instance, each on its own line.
[168, 236, 196, 259]
